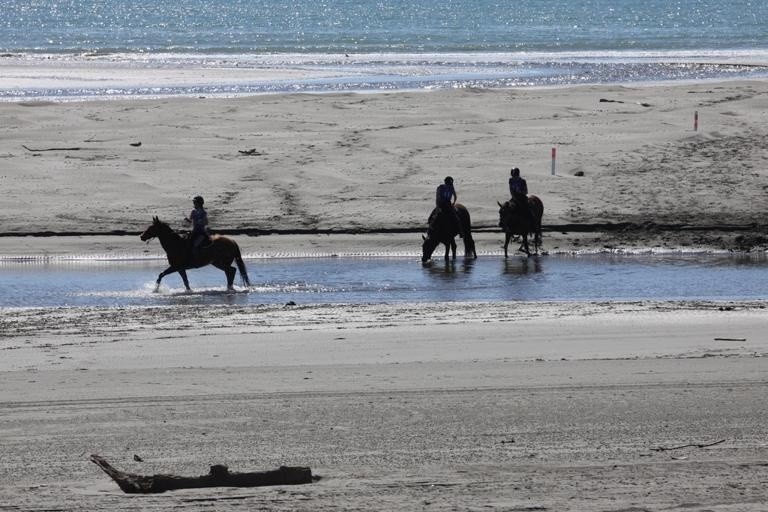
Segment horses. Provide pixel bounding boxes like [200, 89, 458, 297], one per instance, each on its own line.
[140, 215, 250, 294]
[421, 203, 478, 265]
[496, 194, 544, 258]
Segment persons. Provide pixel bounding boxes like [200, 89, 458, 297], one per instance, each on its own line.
[434, 176, 468, 237]
[508, 167, 538, 224]
[185, 196, 212, 255]
